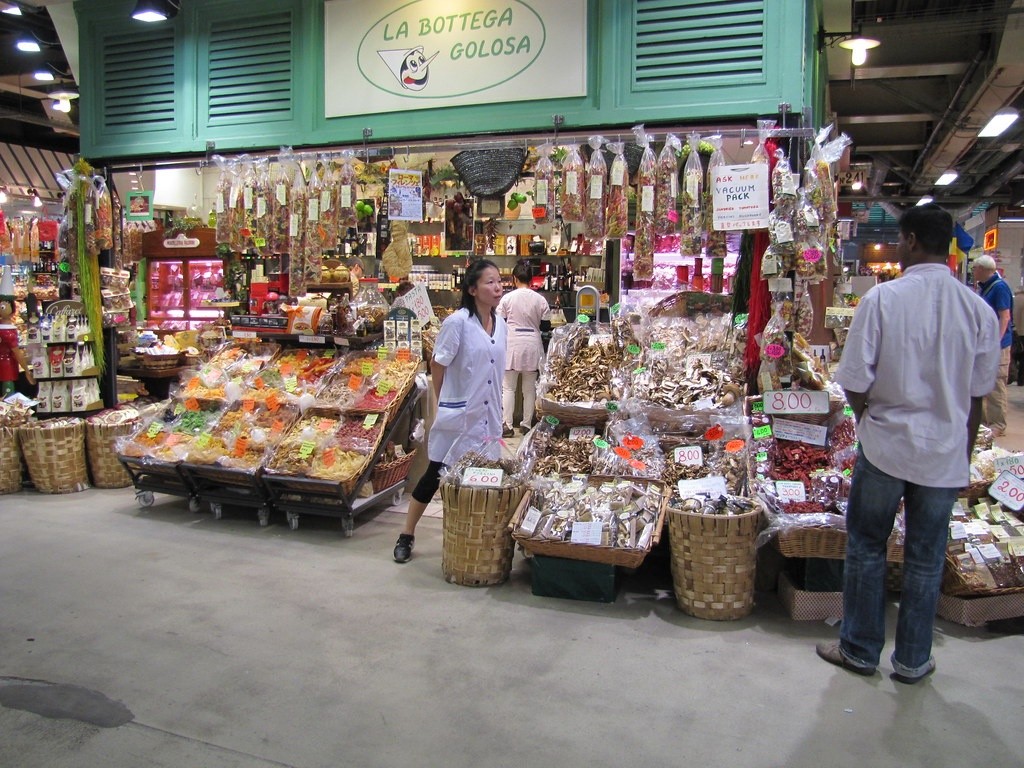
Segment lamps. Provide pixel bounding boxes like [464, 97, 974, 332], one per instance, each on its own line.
[0, 0, 79, 113]
[934, 169, 958, 186]
[916, 195, 933, 207]
[978, 106, 1019, 137]
[818, 23, 882, 66]
[131, 0, 181, 22]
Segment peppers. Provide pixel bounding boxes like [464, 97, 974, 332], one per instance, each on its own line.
[751, 404, 860, 514]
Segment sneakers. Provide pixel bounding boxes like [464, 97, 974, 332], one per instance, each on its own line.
[392, 533, 416, 563]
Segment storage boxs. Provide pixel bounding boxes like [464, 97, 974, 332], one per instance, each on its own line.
[936, 592, 1024, 627]
[530, 554, 618, 603]
[777, 556, 844, 621]
[404, 232, 554, 257]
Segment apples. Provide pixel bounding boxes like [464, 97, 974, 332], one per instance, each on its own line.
[356, 200, 373, 219]
[446, 192, 465, 213]
[507, 193, 527, 210]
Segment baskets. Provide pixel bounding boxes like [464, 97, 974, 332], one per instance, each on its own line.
[0, 426, 26, 496]
[666, 493, 765, 623]
[84, 408, 144, 490]
[259, 405, 388, 498]
[969, 470, 1002, 505]
[181, 398, 303, 493]
[369, 444, 418, 494]
[438, 468, 524, 588]
[18, 415, 92, 495]
[779, 396, 845, 424]
[311, 348, 423, 423]
[504, 473, 672, 569]
[936, 506, 1024, 597]
[749, 482, 900, 564]
[117, 395, 232, 483]
[236, 347, 345, 405]
[176, 338, 282, 403]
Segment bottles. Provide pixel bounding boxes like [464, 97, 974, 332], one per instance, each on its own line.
[544, 263, 585, 291]
[342, 228, 362, 257]
[415, 236, 439, 256]
[499, 268, 518, 292]
[451, 264, 465, 289]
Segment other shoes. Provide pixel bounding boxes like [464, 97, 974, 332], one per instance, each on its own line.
[1007, 374, 1015, 385]
[895, 653, 936, 685]
[502, 425, 514, 438]
[1017, 380, 1024, 386]
[988, 426, 1002, 438]
[518, 424, 530, 435]
[816, 639, 876, 676]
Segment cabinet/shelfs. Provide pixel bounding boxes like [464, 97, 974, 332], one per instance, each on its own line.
[31, 340, 104, 422]
[406, 238, 621, 353]
[30, 236, 61, 300]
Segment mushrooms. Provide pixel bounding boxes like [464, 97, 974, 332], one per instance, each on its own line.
[597, 393, 610, 408]
[721, 383, 740, 405]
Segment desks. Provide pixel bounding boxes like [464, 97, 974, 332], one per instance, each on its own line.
[120, 367, 189, 399]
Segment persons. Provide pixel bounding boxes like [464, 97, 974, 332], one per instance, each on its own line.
[345, 256, 365, 296]
[0, 266, 36, 398]
[392, 259, 508, 563]
[815, 204, 1001, 684]
[396, 281, 415, 296]
[973, 254, 1012, 438]
[496, 260, 551, 437]
